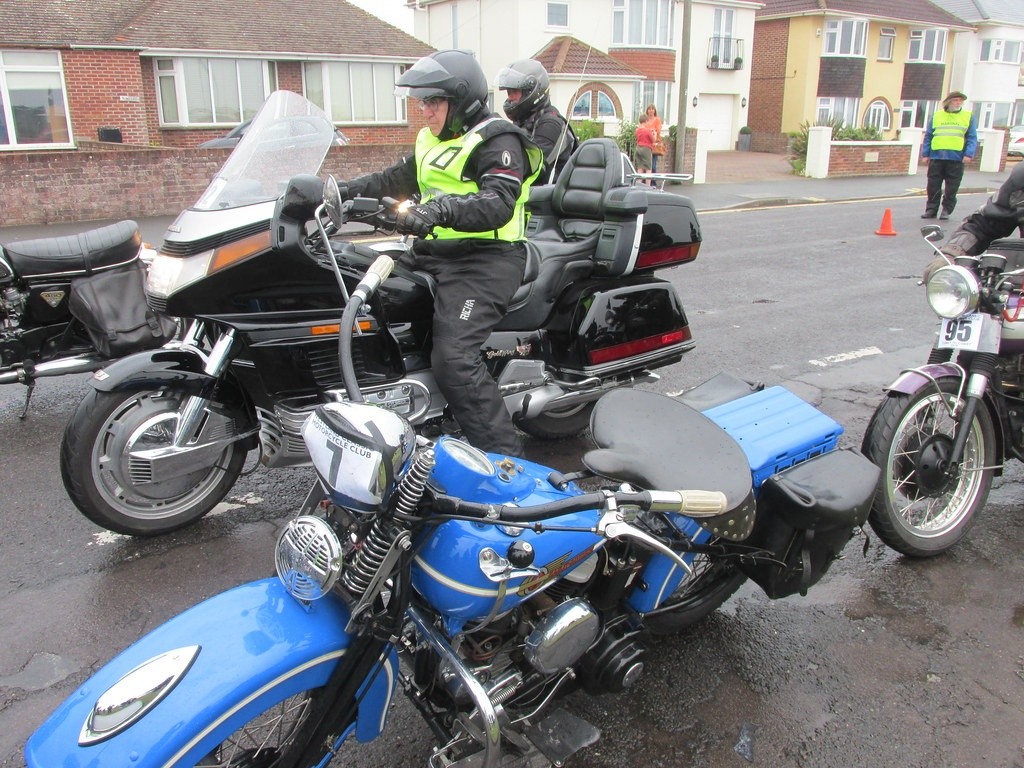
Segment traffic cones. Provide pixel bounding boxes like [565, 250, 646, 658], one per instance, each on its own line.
[874, 208, 897, 236]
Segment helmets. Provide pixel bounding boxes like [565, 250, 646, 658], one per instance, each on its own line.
[396, 49, 488, 133]
[499, 59, 549, 128]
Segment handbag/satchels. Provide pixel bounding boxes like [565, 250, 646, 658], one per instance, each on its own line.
[652, 134, 666, 156]
[738, 443, 882, 599]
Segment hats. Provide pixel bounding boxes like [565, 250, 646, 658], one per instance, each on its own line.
[943, 91, 967, 102]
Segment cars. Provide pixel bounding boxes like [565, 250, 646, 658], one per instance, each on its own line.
[197, 117, 350, 149]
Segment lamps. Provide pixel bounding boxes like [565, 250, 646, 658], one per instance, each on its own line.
[693, 96, 697, 108]
[742, 97, 746, 107]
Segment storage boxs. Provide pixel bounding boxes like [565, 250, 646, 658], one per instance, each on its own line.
[595, 186, 702, 276]
[547, 277, 695, 387]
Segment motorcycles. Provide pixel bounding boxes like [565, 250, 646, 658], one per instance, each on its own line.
[59, 88, 703, 536]
[0, 219, 189, 420]
[861, 225, 1024, 556]
[22, 254, 879, 768]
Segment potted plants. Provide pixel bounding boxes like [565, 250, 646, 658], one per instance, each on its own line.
[734, 57, 743, 69]
[710, 55, 719, 68]
[738, 127, 751, 152]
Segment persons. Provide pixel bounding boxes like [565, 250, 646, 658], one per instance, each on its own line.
[322, 49, 545, 463]
[921, 91, 977, 219]
[634, 104, 662, 190]
[923, 159, 1024, 291]
[499, 58, 578, 187]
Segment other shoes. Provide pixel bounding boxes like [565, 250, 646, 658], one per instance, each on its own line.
[921, 208, 937, 218]
[939, 208, 948, 220]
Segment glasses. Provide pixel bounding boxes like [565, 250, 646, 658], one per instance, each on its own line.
[417, 98, 445, 111]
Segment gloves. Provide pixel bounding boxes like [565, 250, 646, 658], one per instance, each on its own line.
[924, 243, 966, 281]
[396, 200, 442, 240]
